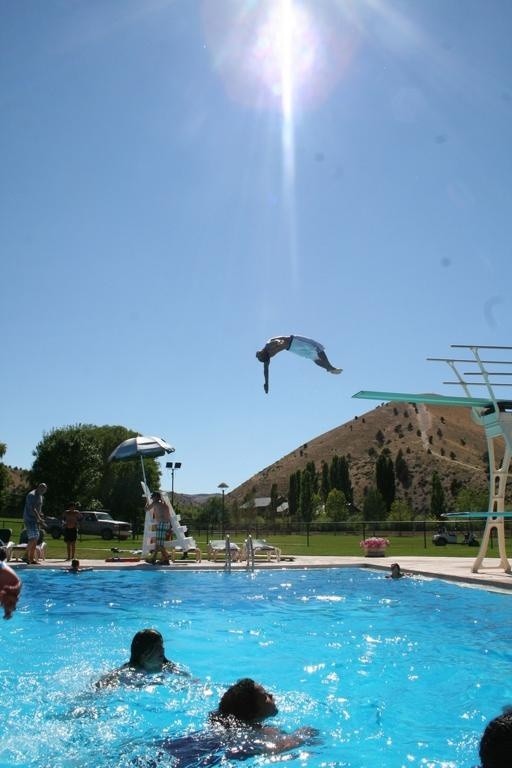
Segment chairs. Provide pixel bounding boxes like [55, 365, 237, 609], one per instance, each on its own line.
[0, 528, 46, 564]
[171, 538, 281, 564]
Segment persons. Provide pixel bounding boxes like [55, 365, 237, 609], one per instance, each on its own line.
[93, 628, 193, 690]
[22, 481, 47, 564]
[61, 557, 91, 572]
[474, 708, 512, 767]
[256, 332, 344, 396]
[133, 677, 319, 768]
[385, 562, 406, 578]
[60, 502, 82, 560]
[143, 491, 171, 566]
[0, 560, 21, 619]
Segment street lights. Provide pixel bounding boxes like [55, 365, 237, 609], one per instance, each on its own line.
[216, 482, 229, 540]
[164, 461, 181, 506]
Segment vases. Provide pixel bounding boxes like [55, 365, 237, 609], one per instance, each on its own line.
[367, 550, 384, 556]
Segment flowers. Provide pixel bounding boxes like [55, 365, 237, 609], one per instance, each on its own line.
[360, 536, 391, 551]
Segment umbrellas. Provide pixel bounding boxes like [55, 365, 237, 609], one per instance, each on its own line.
[107, 433, 176, 497]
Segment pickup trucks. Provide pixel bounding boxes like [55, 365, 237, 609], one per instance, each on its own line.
[46, 510, 134, 541]
